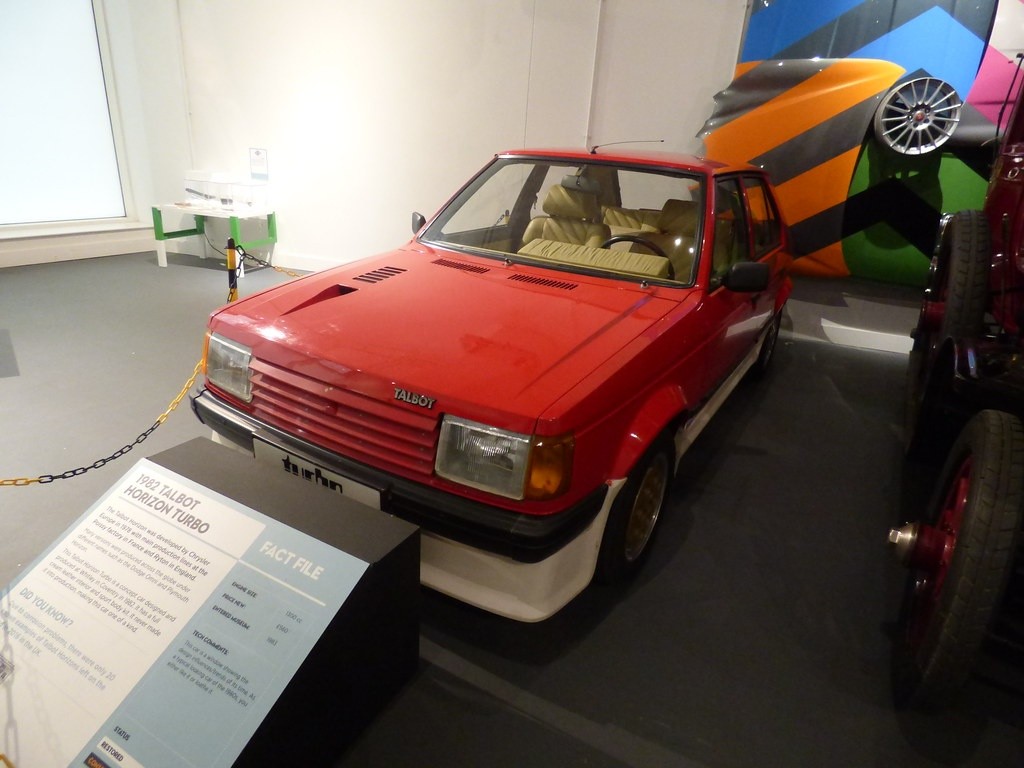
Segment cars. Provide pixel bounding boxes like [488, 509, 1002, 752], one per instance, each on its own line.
[189, 147, 797, 625]
[887, 47, 1023, 716]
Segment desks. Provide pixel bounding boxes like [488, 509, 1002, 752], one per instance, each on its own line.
[151, 202, 277, 277]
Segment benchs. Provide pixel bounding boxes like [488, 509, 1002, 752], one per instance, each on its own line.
[603, 203, 661, 253]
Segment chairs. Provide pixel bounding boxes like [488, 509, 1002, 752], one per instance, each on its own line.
[517, 183, 612, 253]
[628, 199, 729, 282]
